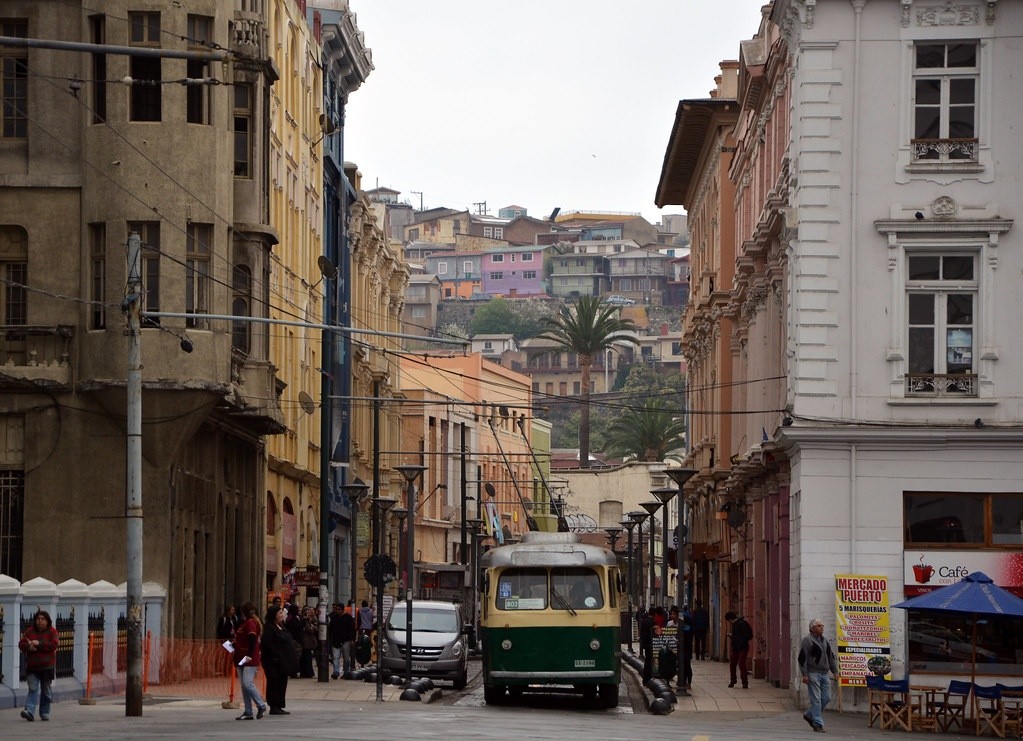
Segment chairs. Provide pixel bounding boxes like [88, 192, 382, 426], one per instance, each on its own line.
[866, 675, 1023, 741]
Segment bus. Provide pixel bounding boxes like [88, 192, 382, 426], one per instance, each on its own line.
[476, 414, 624, 709]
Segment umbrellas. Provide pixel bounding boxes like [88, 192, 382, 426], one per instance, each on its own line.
[890, 571, 1023, 736]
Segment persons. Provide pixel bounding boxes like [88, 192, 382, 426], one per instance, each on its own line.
[260, 596, 317, 715]
[18, 611, 59, 721]
[217, 600, 266, 720]
[326, 599, 374, 679]
[676, 614, 693, 690]
[640, 607, 655, 687]
[724, 611, 754, 689]
[797, 618, 839, 732]
[567, 571, 600, 604]
[635, 599, 710, 660]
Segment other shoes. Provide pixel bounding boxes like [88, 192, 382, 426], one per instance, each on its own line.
[803, 714, 814, 728]
[236, 712, 254, 720]
[331, 674, 338, 679]
[20, 709, 34, 721]
[269, 708, 290, 715]
[814, 725, 825, 733]
[743, 682, 748, 689]
[41, 712, 49, 721]
[256, 705, 266, 719]
[728, 680, 737, 688]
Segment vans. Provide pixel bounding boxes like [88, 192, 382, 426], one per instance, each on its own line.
[381, 599, 473, 691]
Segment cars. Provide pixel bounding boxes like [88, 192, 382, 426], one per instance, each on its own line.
[605, 294, 635, 305]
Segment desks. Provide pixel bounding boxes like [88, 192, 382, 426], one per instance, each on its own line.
[910, 685, 944, 733]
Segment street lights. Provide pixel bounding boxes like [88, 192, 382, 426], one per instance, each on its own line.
[389, 507, 409, 603]
[619, 520, 638, 652]
[605, 527, 623, 554]
[391, 464, 429, 692]
[338, 483, 370, 672]
[649, 487, 679, 629]
[628, 513, 651, 659]
[464, 517, 489, 634]
[638, 500, 665, 610]
[372, 496, 398, 625]
[661, 468, 701, 695]
